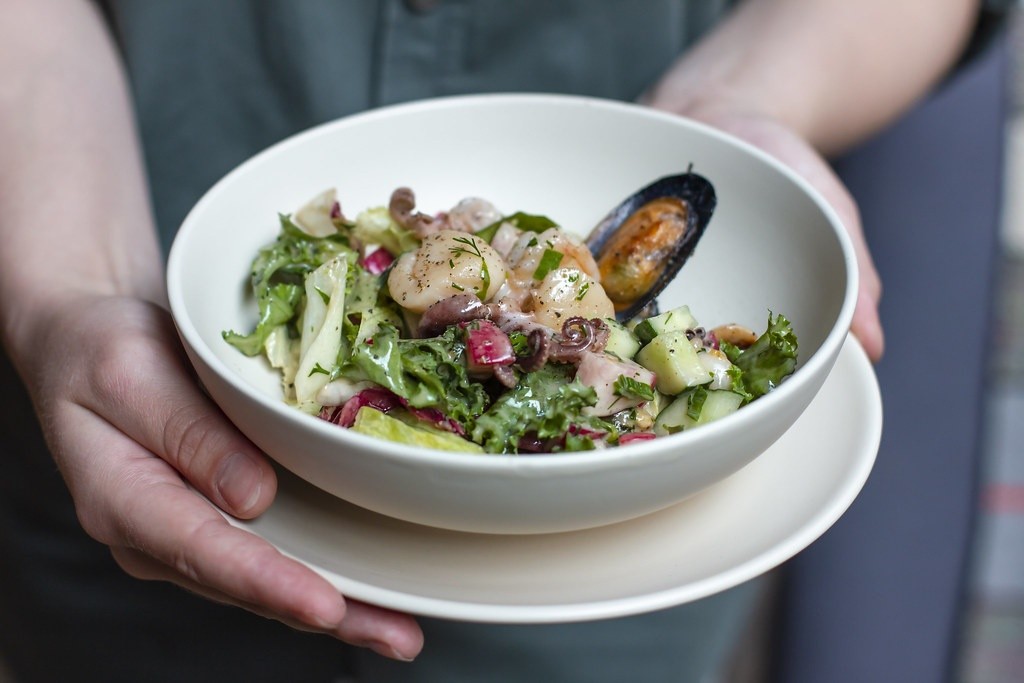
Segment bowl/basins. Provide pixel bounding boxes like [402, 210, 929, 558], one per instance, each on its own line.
[168, 91, 861, 536]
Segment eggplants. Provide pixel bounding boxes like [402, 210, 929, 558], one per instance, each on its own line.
[579, 172, 717, 324]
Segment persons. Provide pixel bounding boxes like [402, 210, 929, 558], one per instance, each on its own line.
[0, 0, 978, 665]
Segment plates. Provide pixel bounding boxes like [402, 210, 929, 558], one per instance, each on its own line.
[184, 328, 882, 625]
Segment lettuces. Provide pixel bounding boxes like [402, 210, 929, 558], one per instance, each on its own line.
[215, 213, 802, 458]
